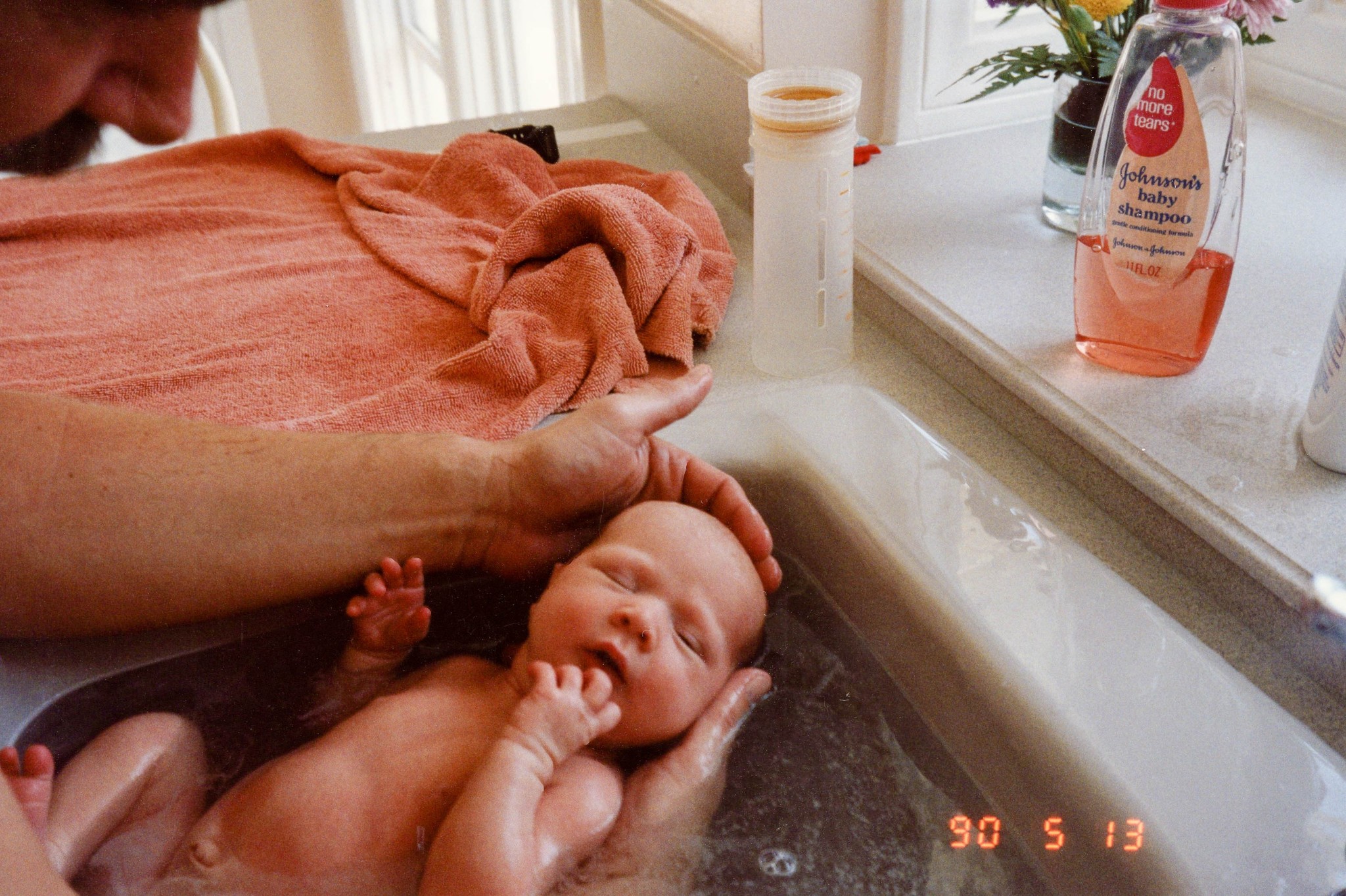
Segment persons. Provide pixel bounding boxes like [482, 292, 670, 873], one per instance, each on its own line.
[1, 0, 785, 896]
[1, 502, 769, 895]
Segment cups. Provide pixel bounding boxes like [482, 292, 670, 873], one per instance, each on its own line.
[745, 66, 860, 380]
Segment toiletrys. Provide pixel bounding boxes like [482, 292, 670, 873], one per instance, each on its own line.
[1071, 0, 1252, 379]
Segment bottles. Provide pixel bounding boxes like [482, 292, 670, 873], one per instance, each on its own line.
[1300, 263, 1345, 474]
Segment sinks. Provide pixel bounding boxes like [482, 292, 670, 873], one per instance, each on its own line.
[4, 418, 1339, 888]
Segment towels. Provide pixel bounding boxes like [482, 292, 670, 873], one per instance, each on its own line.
[2, 125, 738, 431]
